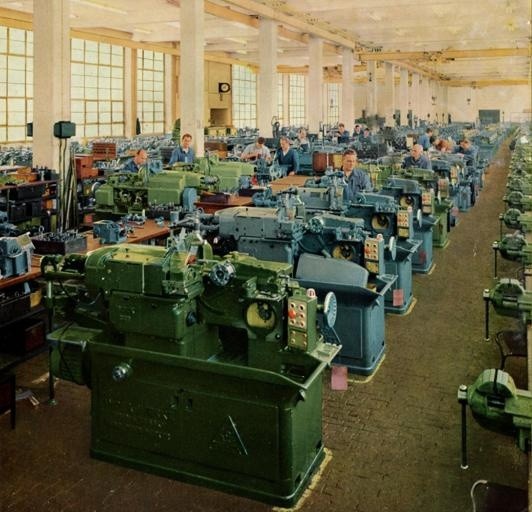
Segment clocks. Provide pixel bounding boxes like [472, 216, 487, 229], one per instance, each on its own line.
[219, 82, 230, 93]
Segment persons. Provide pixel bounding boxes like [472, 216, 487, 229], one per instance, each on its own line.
[430, 136, 451, 154]
[401, 144, 433, 171]
[416, 128, 433, 150]
[119, 148, 148, 177]
[359, 128, 370, 143]
[241, 137, 273, 161]
[353, 125, 364, 138]
[339, 149, 370, 194]
[292, 127, 311, 149]
[454, 137, 475, 172]
[337, 124, 349, 142]
[169, 134, 194, 164]
[272, 137, 300, 176]
